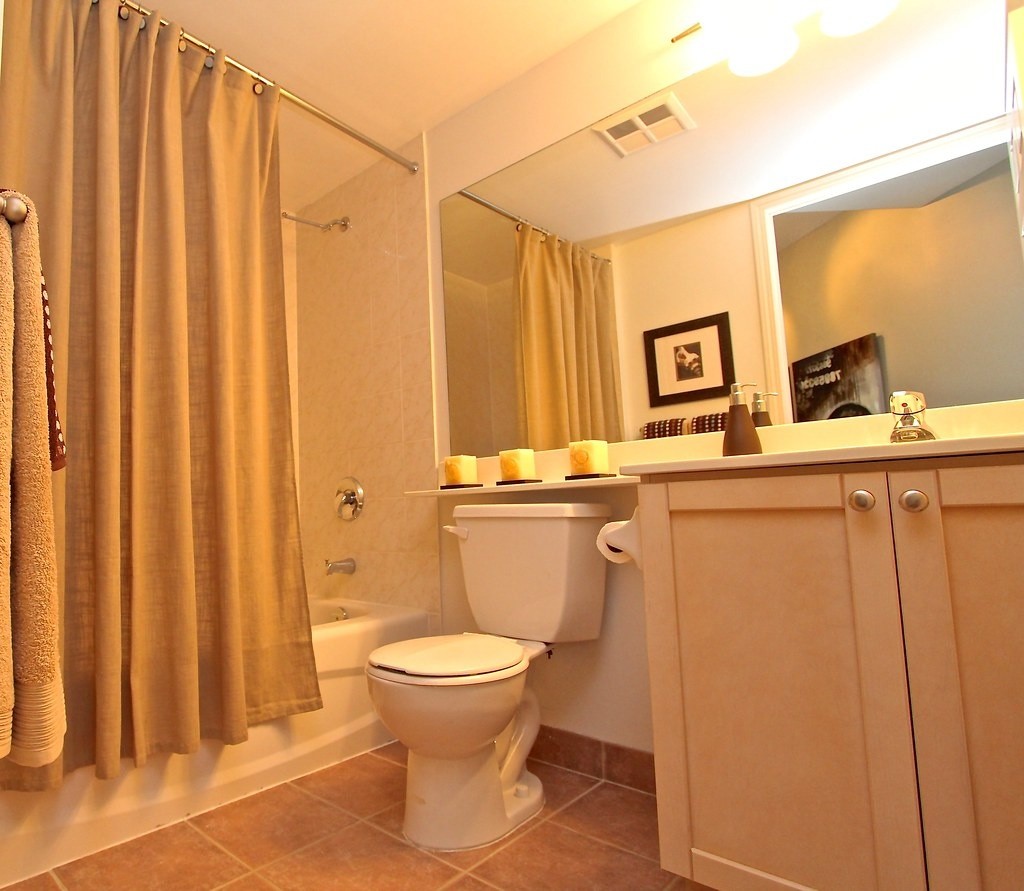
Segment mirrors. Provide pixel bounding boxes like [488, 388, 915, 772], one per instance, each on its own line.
[417, 1, 1024, 485]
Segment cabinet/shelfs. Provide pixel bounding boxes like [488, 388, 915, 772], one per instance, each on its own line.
[630, 451, 1024, 891]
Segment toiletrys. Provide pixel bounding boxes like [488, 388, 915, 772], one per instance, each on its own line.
[719, 383, 767, 457]
[749, 389, 780, 429]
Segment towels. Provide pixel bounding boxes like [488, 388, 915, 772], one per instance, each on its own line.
[0, 185, 71, 474]
[639, 408, 734, 441]
[1, 188, 72, 771]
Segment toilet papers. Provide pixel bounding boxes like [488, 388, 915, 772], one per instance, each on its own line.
[593, 517, 635, 566]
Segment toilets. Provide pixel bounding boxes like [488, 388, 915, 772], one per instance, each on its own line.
[362, 500, 612, 855]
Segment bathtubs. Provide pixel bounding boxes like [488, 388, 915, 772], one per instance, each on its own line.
[1, 594, 433, 891]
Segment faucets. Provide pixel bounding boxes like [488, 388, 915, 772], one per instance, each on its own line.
[324, 554, 357, 578]
[885, 387, 939, 444]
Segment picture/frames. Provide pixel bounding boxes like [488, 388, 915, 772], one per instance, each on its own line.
[644, 312, 736, 408]
